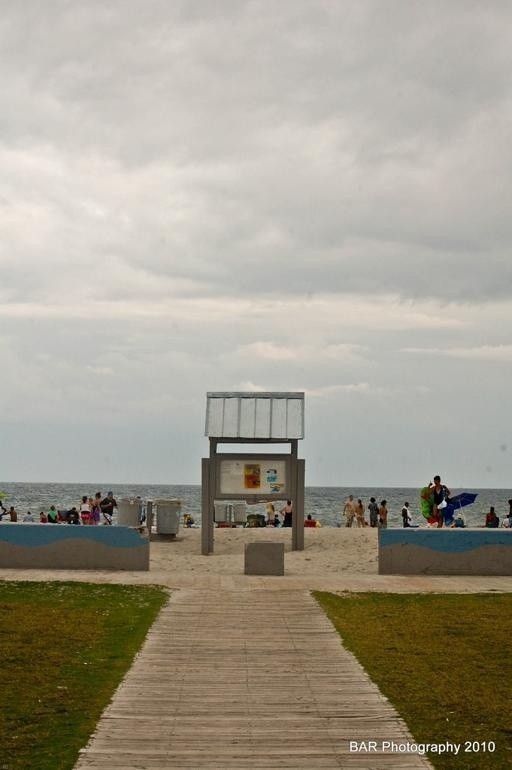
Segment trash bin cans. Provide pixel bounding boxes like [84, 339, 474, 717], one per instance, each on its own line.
[215, 502, 248, 528]
[117, 497, 180, 541]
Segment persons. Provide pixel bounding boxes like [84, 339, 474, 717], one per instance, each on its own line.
[265, 502, 276, 521]
[136, 496, 145, 533]
[279, 500, 293, 527]
[0, 491, 118, 525]
[274, 514, 282, 527]
[307, 514, 311, 520]
[343, 476, 512, 528]
[185, 513, 192, 528]
